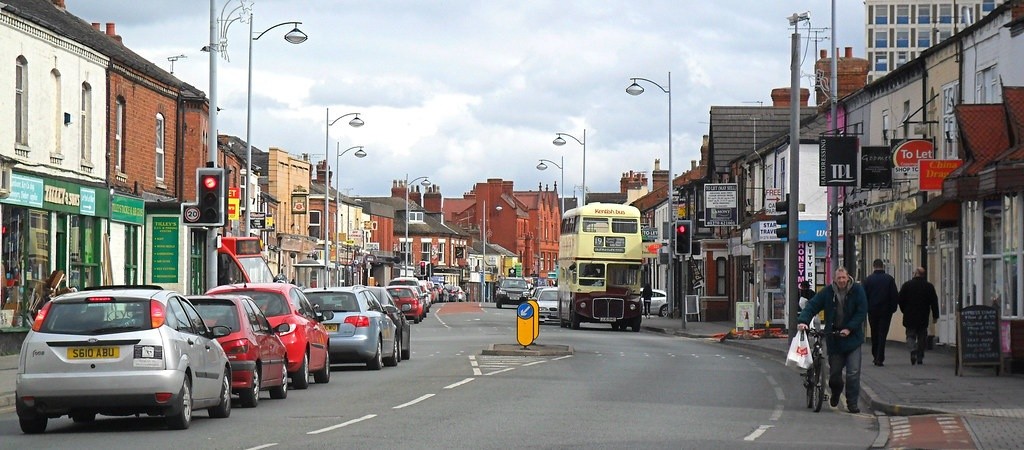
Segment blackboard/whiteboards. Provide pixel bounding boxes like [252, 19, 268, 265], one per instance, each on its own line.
[956, 305, 1003, 365]
[685, 295, 700, 314]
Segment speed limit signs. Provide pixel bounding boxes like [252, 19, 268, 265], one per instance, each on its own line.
[185, 207, 200, 222]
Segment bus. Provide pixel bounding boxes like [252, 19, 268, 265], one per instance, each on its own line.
[556, 203, 648, 333]
[218, 237, 274, 285]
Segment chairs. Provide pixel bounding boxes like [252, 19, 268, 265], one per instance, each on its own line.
[342, 299, 356, 311]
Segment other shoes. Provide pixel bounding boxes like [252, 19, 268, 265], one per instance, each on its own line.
[911, 349, 918, 365]
[917, 358, 923, 364]
[830, 391, 841, 407]
[645, 315, 647, 319]
[819, 348, 822, 355]
[873, 356, 884, 366]
[851, 405, 860, 413]
[647, 313, 650, 319]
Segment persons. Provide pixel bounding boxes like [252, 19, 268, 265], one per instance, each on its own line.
[443, 288, 449, 302]
[263, 274, 289, 316]
[580, 266, 598, 286]
[899, 267, 940, 365]
[861, 259, 898, 367]
[642, 284, 652, 319]
[374, 281, 381, 286]
[465, 290, 469, 302]
[797, 267, 868, 414]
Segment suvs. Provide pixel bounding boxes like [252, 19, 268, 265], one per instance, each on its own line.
[495, 277, 530, 308]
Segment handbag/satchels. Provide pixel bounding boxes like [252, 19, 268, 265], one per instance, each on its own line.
[784, 329, 814, 375]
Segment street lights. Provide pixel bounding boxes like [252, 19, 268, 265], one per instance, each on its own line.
[537, 157, 564, 218]
[553, 130, 586, 206]
[625, 73, 673, 317]
[324, 108, 364, 289]
[246, 14, 308, 237]
[404, 174, 431, 276]
[334, 142, 367, 292]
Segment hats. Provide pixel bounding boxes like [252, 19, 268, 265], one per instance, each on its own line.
[800, 281, 810, 286]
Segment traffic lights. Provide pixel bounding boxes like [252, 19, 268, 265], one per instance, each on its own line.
[675, 220, 693, 255]
[199, 170, 220, 222]
[775, 193, 789, 242]
[420, 261, 426, 276]
[508, 268, 516, 277]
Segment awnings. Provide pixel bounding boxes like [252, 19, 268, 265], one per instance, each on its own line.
[905, 194, 962, 224]
[942, 159, 1024, 201]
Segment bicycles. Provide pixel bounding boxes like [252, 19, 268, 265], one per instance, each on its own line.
[800, 328, 844, 412]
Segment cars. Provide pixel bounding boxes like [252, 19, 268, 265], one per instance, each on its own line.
[385, 278, 467, 324]
[15, 285, 411, 435]
[527, 286, 560, 324]
